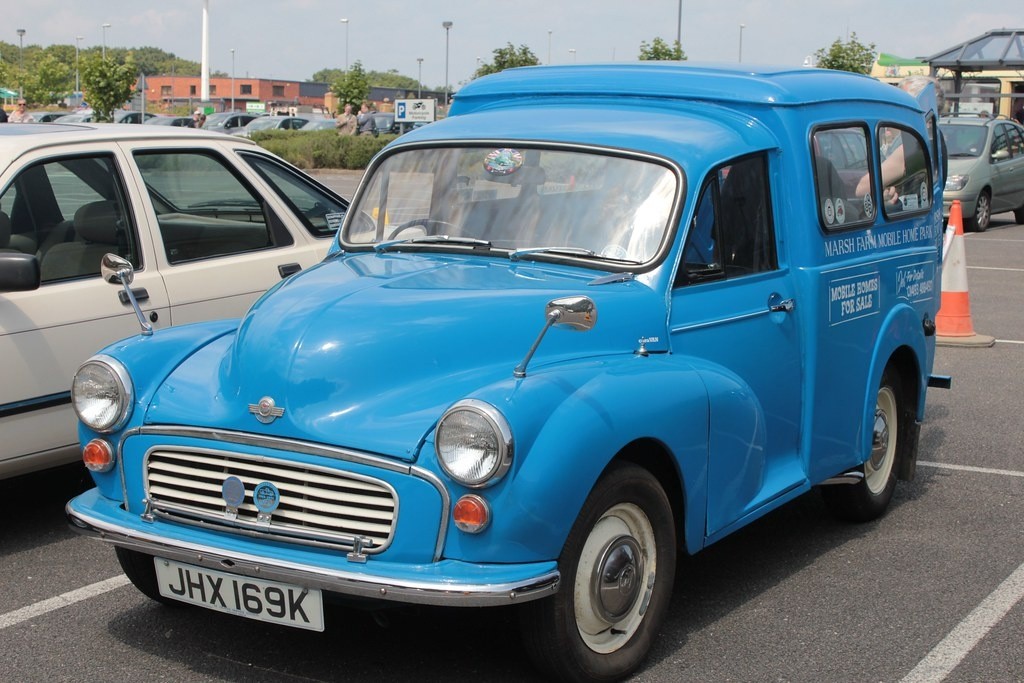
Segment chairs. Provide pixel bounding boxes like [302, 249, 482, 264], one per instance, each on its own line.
[0, 210, 24, 252]
[39, 200, 121, 279]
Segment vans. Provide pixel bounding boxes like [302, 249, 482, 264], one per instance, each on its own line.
[949, 82, 1002, 118]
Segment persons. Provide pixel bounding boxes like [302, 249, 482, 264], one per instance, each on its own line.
[855, 74, 948, 196]
[335, 103, 376, 135]
[8, 100, 35, 123]
[815, 156, 898, 205]
[187, 111, 208, 130]
[58, 98, 68, 108]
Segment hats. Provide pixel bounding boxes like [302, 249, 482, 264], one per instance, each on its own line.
[194, 111, 200, 114]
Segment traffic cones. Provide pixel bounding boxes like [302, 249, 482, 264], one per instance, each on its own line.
[933, 198, 996, 347]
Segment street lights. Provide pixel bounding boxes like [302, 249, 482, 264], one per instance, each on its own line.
[17, 29, 26, 98]
[739, 25, 745, 61]
[416, 59, 423, 99]
[230, 48, 237, 112]
[102, 24, 111, 59]
[341, 18, 349, 81]
[76, 36, 83, 107]
[441, 22, 453, 112]
[547, 28, 553, 64]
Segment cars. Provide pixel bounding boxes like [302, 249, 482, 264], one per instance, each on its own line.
[28, 111, 425, 138]
[66, 60, 956, 682]
[812, 130, 883, 175]
[885, 117, 1024, 232]
[1, 119, 419, 483]
